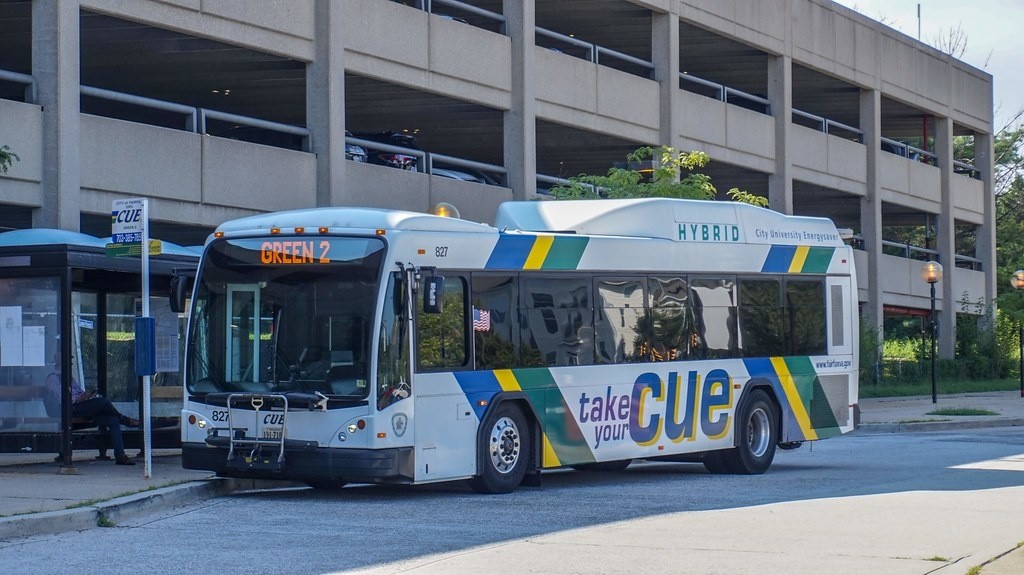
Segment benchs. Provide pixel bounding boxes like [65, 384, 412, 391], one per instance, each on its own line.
[22, 416, 181, 462]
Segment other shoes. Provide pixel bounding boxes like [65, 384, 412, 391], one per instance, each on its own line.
[120, 416, 140, 427]
[116, 454, 135, 465]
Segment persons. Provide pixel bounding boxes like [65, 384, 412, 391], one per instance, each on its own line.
[43, 350, 143, 465]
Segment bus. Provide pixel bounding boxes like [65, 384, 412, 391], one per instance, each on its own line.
[170, 196, 860, 496]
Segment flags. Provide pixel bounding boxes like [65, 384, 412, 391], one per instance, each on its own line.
[473, 308, 491, 331]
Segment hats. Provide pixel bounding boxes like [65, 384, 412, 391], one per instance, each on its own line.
[53, 352, 74, 364]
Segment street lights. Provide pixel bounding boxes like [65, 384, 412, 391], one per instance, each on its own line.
[1010, 269, 1024, 398]
[922, 260, 943, 404]
